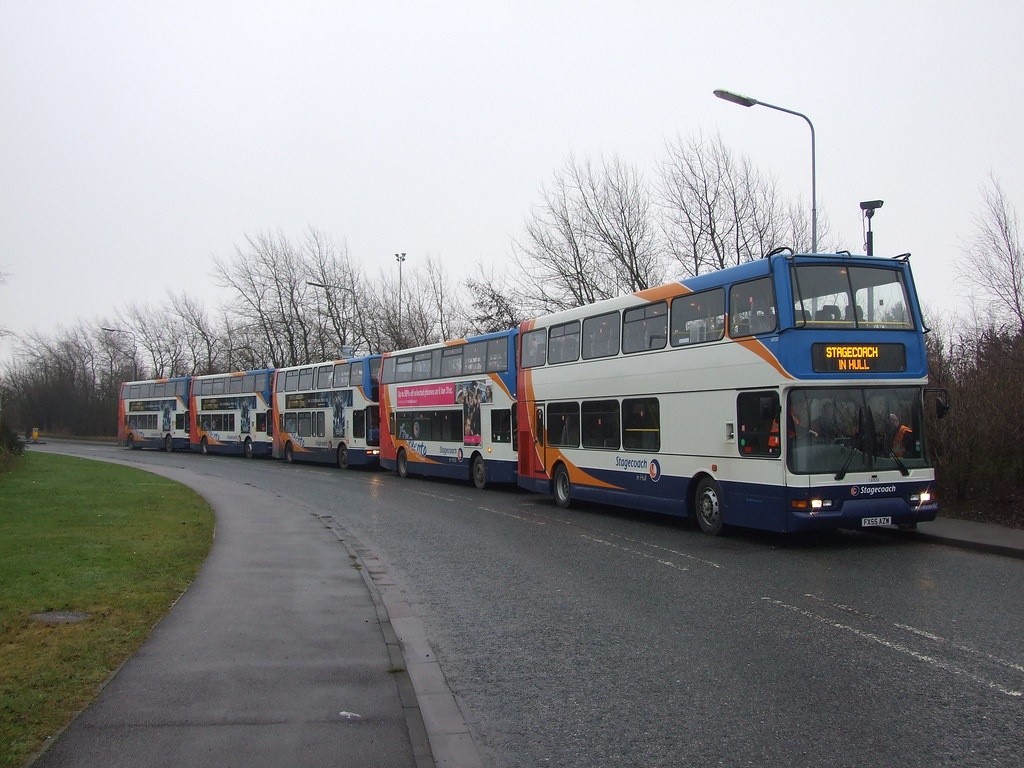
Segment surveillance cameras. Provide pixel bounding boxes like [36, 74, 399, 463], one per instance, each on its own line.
[860, 200, 884, 210]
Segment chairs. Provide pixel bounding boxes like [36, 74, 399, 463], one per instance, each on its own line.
[534, 344, 545, 365]
[625, 323, 644, 352]
[549, 416, 656, 450]
[591, 330, 608, 358]
[607, 326, 618, 355]
[549, 341, 562, 364]
[644, 318, 665, 349]
[689, 304, 866, 342]
[576, 334, 592, 359]
[561, 339, 577, 362]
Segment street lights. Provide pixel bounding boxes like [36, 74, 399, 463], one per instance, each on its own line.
[713, 87, 817, 254]
[306, 281, 356, 357]
[101, 327, 135, 382]
[395, 252, 406, 350]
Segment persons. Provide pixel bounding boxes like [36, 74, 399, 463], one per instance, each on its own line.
[810, 402, 846, 439]
[767, 399, 818, 453]
[455, 385, 491, 436]
[889, 414, 917, 529]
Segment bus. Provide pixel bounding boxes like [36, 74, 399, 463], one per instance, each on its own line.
[270, 353, 383, 469]
[190, 367, 279, 459]
[378, 325, 517, 490]
[517, 246, 952, 538]
[117, 376, 196, 452]
